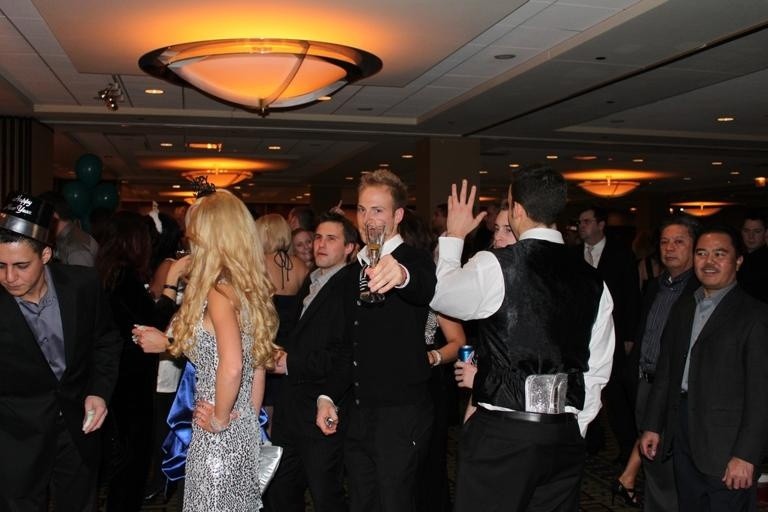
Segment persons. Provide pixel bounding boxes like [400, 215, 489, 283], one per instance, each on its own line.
[0, 159, 768, 510]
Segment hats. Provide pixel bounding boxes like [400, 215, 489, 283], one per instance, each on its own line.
[0, 193, 57, 246]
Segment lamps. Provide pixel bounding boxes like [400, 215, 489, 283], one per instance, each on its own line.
[573, 179, 640, 197]
[138, 37, 383, 116]
[183, 197, 198, 204]
[182, 167, 255, 188]
[678, 206, 725, 218]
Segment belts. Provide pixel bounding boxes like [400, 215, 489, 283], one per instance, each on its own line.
[637, 371, 655, 383]
[506, 411, 575, 423]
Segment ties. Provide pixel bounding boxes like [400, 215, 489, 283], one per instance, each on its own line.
[584, 246, 594, 266]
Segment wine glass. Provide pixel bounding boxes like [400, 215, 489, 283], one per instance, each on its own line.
[359, 221, 386, 304]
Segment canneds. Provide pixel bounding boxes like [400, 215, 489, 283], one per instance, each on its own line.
[459, 345, 476, 366]
